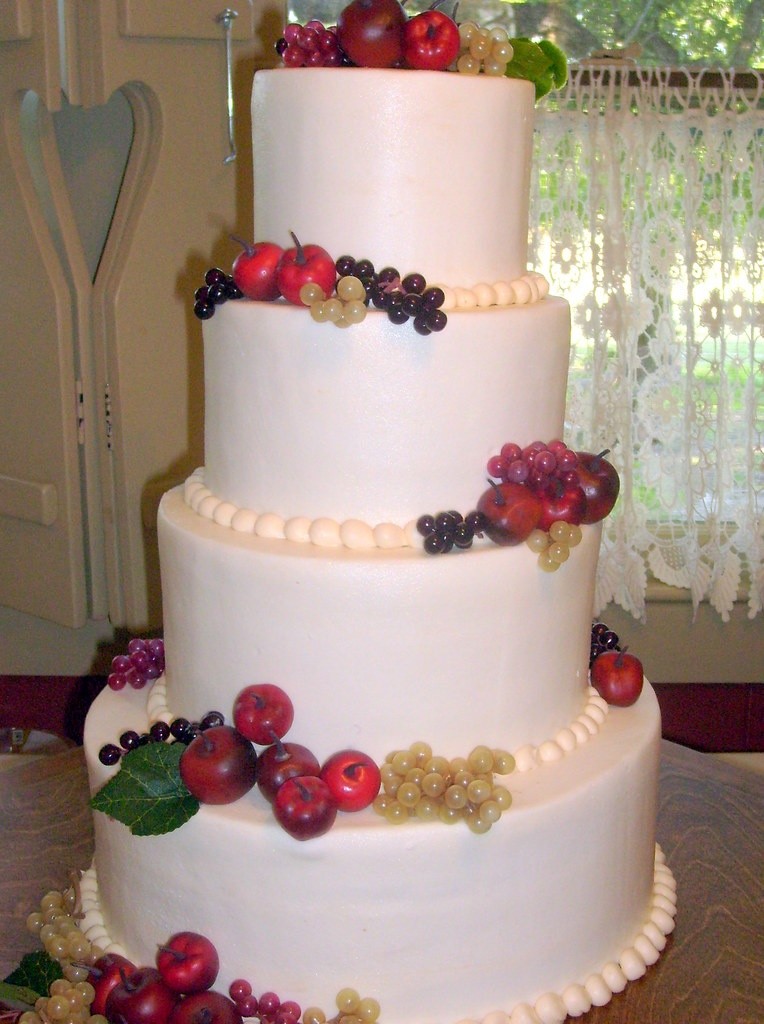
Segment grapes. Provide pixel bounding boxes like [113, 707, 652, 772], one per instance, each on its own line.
[27, 889, 102, 985]
[17, 979, 109, 1024]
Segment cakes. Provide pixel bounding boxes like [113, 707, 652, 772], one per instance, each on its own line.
[68, 0, 678, 1024]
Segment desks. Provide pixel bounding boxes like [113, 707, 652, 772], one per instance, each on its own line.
[0, 734, 764, 1024]
